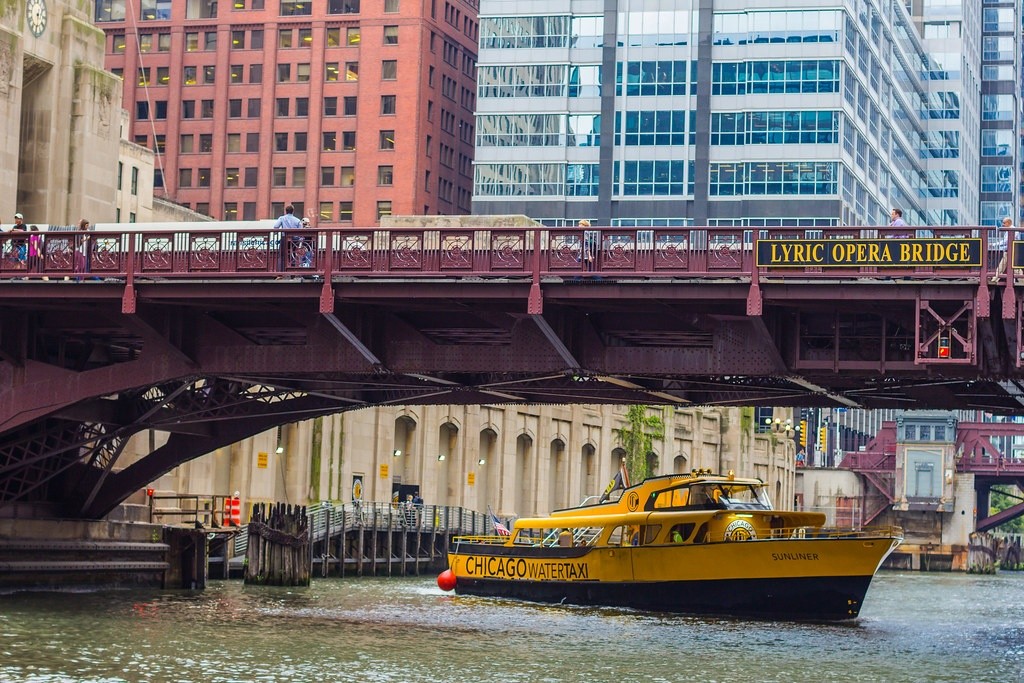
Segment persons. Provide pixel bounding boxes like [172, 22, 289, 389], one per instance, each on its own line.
[579, 540, 587, 546]
[74, 218, 90, 280]
[670, 524, 683, 542]
[405, 491, 423, 527]
[989, 218, 1023, 283]
[796, 449, 805, 467]
[558, 528, 574, 548]
[574, 219, 596, 272]
[273, 205, 312, 280]
[0, 213, 44, 281]
[887, 208, 911, 239]
[478, 540, 485, 544]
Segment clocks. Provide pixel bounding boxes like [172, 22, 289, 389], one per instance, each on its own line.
[26, 0, 47, 37]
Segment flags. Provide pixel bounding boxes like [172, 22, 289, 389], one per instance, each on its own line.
[491, 514, 511, 536]
[599, 463, 631, 504]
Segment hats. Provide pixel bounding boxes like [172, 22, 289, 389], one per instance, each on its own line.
[14, 213, 23, 219]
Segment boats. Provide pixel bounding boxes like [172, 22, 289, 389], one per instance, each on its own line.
[447, 468, 904, 627]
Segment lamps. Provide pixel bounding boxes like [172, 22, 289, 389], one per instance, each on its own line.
[439, 454, 445, 460]
[479, 459, 485, 464]
[275, 447, 283, 454]
[394, 450, 401, 456]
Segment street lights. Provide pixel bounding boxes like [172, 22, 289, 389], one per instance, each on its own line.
[765, 418, 801, 437]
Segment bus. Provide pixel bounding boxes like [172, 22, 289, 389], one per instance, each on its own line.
[0, 219, 280, 259]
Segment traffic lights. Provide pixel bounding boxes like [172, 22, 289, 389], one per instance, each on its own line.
[820, 427, 826, 453]
[801, 407, 808, 421]
[800, 420, 806, 447]
